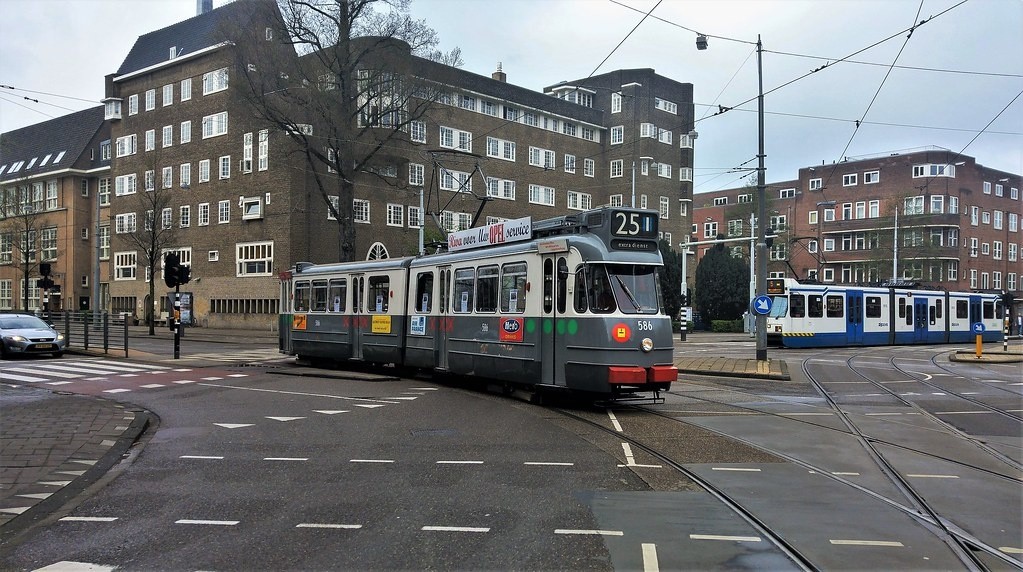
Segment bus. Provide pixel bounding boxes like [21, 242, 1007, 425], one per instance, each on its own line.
[766, 275, 1006, 350]
[277, 207, 680, 412]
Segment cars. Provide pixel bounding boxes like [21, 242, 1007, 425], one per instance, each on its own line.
[0, 314, 65, 358]
